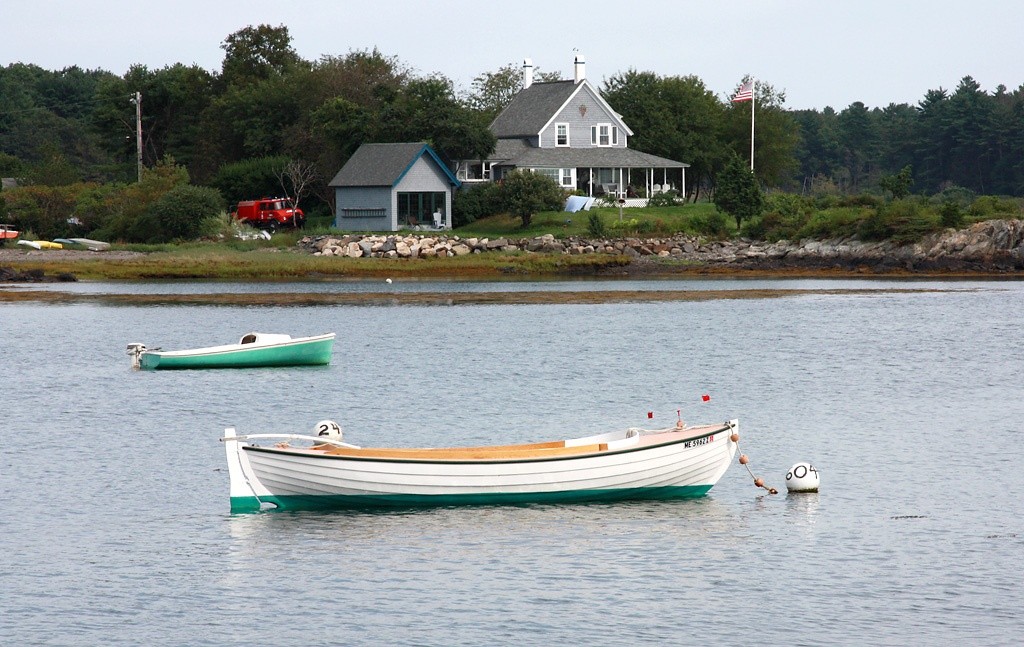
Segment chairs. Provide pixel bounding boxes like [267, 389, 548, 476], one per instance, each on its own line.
[608, 185, 627, 198]
[407, 217, 421, 229]
[433, 213, 446, 229]
[653, 184, 670, 194]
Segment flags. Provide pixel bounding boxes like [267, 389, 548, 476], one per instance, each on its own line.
[730, 81, 753, 102]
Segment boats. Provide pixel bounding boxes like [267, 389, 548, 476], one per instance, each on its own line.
[126, 331, 336, 371]
[0, 223, 111, 251]
[219, 407, 739, 516]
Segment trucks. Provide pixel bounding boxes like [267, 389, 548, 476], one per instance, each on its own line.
[231, 197, 304, 235]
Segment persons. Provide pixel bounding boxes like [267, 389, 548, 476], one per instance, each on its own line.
[627, 183, 636, 198]
